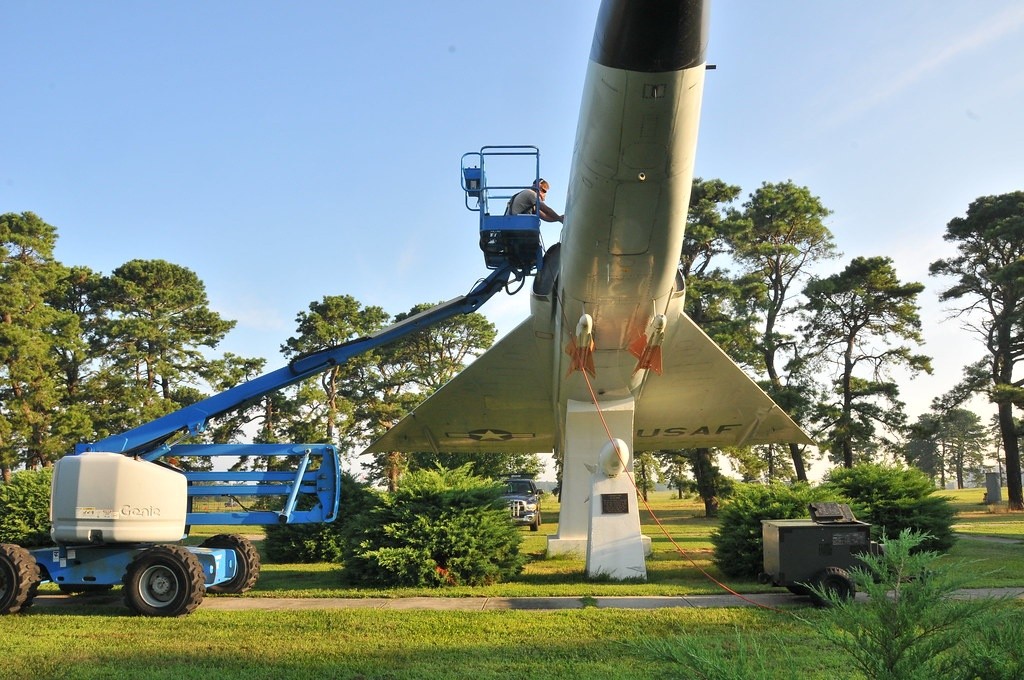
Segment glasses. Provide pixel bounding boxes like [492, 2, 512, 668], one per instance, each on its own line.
[541, 187, 547, 192]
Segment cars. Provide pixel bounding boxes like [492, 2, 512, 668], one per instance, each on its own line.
[496, 477, 541, 531]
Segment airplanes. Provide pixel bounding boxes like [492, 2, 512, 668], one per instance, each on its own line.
[360, 2, 817, 581]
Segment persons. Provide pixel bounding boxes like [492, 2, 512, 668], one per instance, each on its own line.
[505, 179, 564, 224]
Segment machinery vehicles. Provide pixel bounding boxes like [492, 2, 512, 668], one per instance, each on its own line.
[1, 145, 547, 616]
